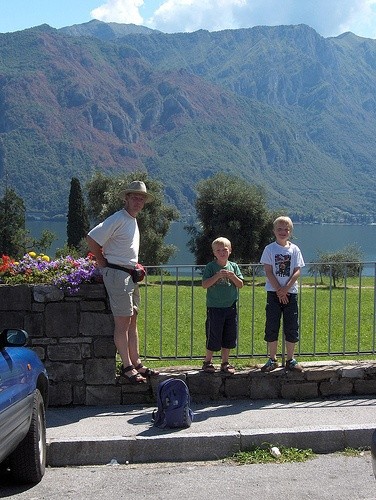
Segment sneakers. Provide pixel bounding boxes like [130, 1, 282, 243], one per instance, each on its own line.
[221, 362, 236, 373]
[286, 358, 303, 372]
[202, 361, 215, 373]
[261, 358, 279, 372]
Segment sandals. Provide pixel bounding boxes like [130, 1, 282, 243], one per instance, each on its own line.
[134, 363, 160, 377]
[118, 364, 147, 384]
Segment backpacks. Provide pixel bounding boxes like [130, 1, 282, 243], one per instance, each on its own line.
[151, 378, 194, 429]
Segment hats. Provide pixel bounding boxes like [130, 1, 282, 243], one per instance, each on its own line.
[118, 181, 155, 203]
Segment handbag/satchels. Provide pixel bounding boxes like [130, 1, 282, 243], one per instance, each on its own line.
[128, 264, 147, 282]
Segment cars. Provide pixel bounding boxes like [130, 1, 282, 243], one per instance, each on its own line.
[0, 328, 49, 483]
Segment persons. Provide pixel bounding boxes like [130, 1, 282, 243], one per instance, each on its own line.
[87, 181, 159, 385]
[201, 237, 245, 373]
[260, 216, 305, 371]
